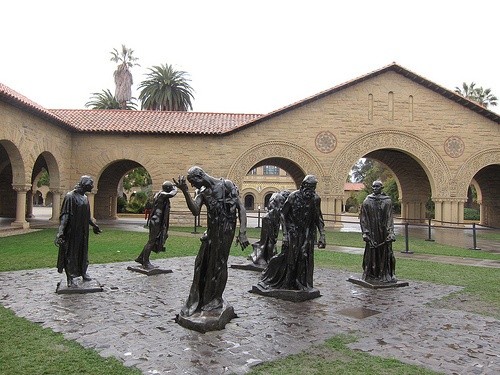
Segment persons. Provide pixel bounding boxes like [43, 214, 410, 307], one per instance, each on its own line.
[56, 175, 103, 288]
[172, 165, 249, 312]
[249, 190, 289, 264]
[259, 175, 326, 290]
[359, 181, 396, 283]
[134, 180, 178, 269]
